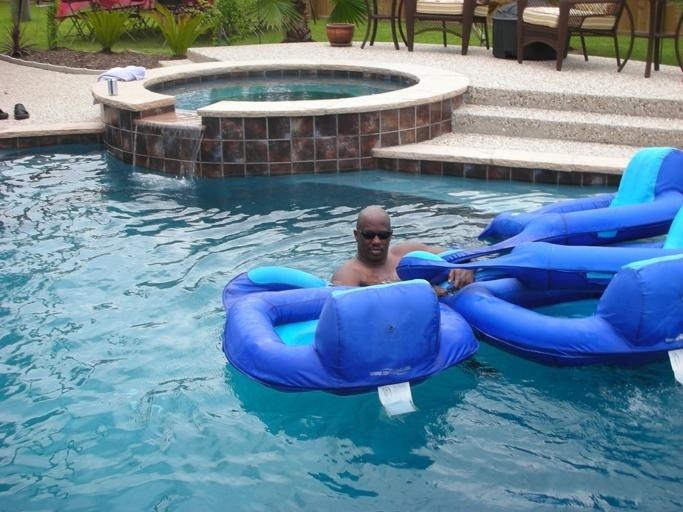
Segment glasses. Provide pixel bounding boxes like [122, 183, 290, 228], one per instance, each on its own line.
[357, 227, 393, 240]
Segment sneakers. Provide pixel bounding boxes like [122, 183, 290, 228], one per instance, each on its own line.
[0, 108, 8, 119]
[14, 103, 29, 119]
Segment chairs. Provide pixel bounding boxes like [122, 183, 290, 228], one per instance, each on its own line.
[404, 0, 626, 73]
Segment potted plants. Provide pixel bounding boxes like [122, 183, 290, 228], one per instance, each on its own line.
[326, 23, 355, 48]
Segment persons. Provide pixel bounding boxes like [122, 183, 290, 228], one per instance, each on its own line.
[330, 205, 475, 296]
